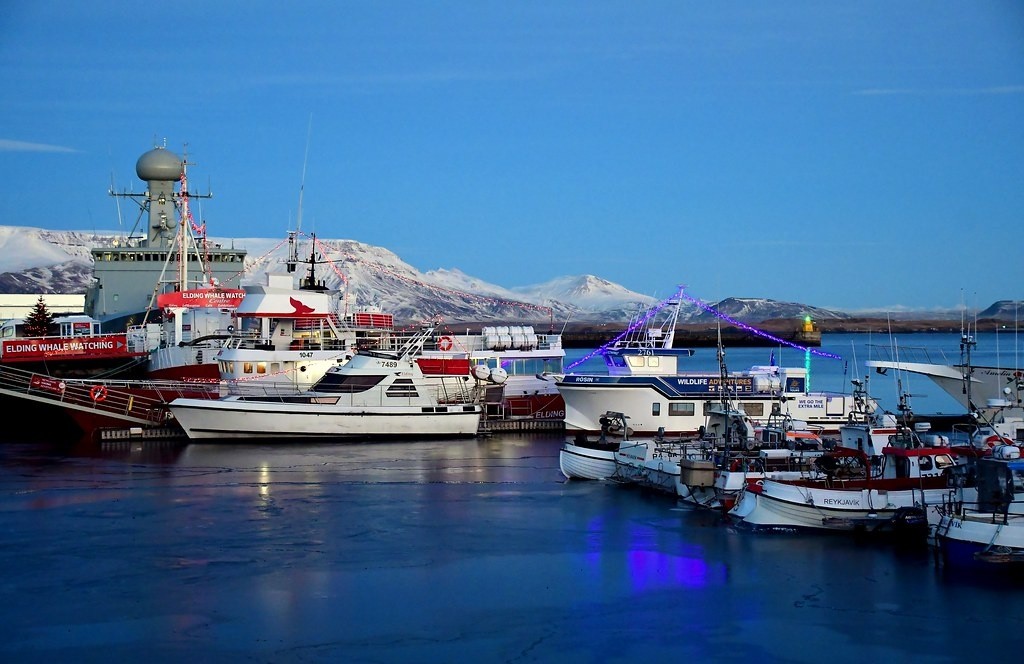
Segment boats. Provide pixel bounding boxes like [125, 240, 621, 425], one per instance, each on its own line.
[862, 287, 1024, 424]
[0, 232, 567, 442]
[611, 304, 830, 483]
[713, 375, 925, 512]
[554, 285, 877, 480]
[165, 349, 483, 444]
[728, 379, 1021, 526]
[934, 458, 1024, 570]
[643, 435, 842, 494]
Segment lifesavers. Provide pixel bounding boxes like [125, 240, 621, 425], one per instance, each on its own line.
[89, 385, 108, 403]
[437, 337, 452, 350]
[986, 435, 1014, 456]
